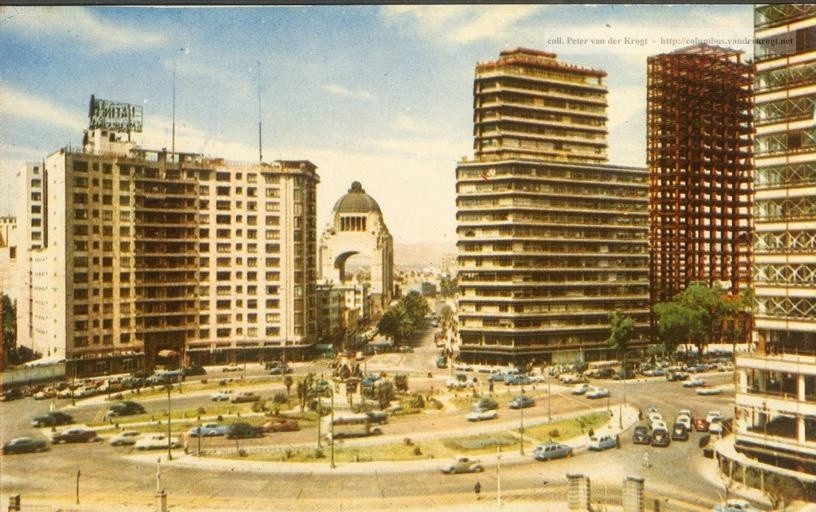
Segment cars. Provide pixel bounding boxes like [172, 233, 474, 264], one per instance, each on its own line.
[637, 353, 735, 381]
[714, 499, 765, 512]
[534, 441, 575, 462]
[474, 393, 499, 412]
[467, 406, 497, 422]
[355, 351, 364, 362]
[361, 373, 384, 387]
[7, 401, 299, 458]
[445, 374, 473, 387]
[211, 358, 293, 404]
[426, 308, 448, 369]
[508, 394, 534, 411]
[0, 365, 209, 401]
[633, 405, 724, 448]
[455, 365, 478, 376]
[478, 367, 500, 376]
[697, 387, 721, 396]
[588, 433, 616, 451]
[441, 453, 482, 474]
[488, 370, 610, 399]
[363, 410, 389, 425]
[683, 378, 706, 387]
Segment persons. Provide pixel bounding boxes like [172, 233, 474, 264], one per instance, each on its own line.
[589, 428, 594, 437]
[643, 450, 651, 468]
[474, 481, 481, 499]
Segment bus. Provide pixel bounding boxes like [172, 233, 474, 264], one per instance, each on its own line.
[582, 359, 622, 375]
[324, 411, 369, 438]
